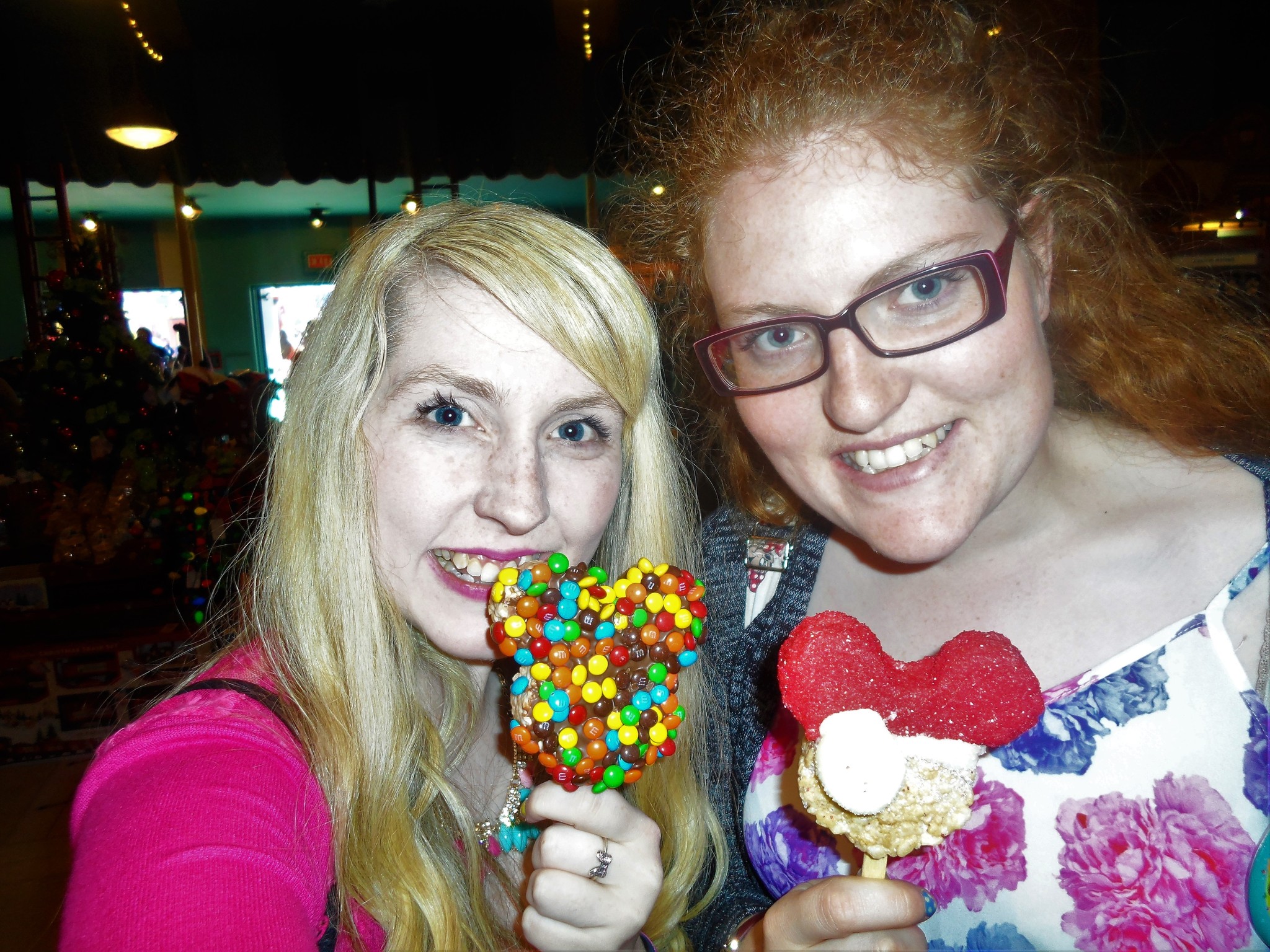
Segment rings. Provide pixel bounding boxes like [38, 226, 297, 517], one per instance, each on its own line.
[589, 837, 612, 882]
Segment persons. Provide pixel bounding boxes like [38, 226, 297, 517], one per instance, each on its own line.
[605, 0, 1270, 950]
[59, 196, 729, 951]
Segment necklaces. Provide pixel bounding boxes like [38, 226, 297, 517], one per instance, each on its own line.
[417, 662, 541, 880]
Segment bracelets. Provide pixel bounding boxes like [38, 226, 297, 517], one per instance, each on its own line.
[722, 912, 769, 952]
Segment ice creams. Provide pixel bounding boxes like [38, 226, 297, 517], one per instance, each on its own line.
[772, 606, 1042, 865]
[485, 548, 710, 800]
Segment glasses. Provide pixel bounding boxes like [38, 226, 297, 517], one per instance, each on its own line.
[694, 217, 1018, 399]
